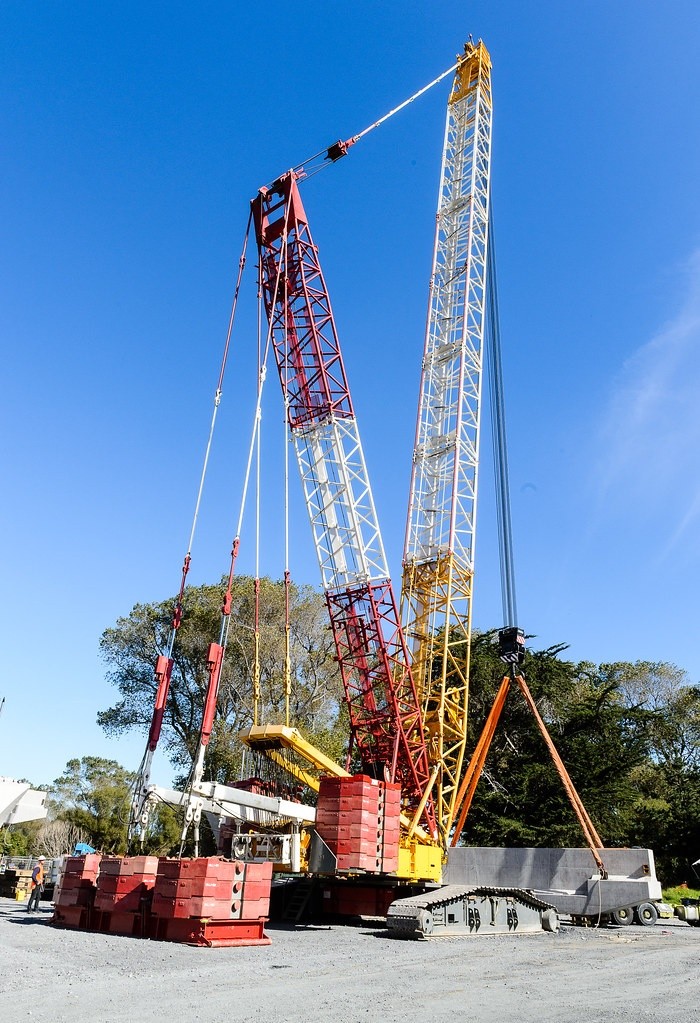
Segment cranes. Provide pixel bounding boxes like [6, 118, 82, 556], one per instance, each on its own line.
[103, 37, 569, 942]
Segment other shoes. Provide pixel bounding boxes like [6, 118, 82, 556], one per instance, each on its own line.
[35, 910, 42, 913]
[28, 910, 31, 913]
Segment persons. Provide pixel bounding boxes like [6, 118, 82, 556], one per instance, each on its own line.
[27, 855, 48, 914]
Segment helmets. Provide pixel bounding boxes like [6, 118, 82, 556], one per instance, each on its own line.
[38, 855, 46, 860]
